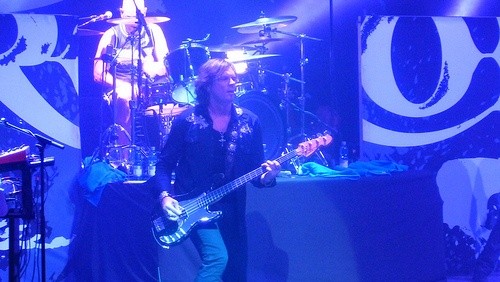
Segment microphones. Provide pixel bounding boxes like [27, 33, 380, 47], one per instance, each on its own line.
[80, 12, 113, 28]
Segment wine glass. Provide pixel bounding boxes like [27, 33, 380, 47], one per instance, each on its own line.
[106, 144, 124, 168]
[121, 145, 137, 175]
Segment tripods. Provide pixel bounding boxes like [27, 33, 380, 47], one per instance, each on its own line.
[272, 33, 331, 170]
[83, 23, 155, 178]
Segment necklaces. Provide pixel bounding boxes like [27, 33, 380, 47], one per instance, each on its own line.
[212, 113, 230, 147]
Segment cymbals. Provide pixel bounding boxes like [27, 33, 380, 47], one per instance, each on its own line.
[240, 37, 290, 45]
[78, 28, 106, 36]
[231, 16, 297, 34]
[224, 54, 282, 63]
[202, 42, 258, 53]
[106, 16, 171, 25]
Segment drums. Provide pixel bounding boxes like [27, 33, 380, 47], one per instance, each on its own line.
[232, 89, 287, 162]
[163, 42, 212, 105]
[140, 75, 193, 118]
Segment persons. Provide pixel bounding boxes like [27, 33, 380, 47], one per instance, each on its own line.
[154, 59, 281, 282]
[94, 0, 170, 162]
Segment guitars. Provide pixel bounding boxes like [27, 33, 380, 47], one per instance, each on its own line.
[149, 129, 334, 251]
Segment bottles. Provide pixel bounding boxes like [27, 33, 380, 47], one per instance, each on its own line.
[262, 143, 270, 162]
[339, 141, 348, 169]
[133, 147, 143, 180]
[147, 146, 158, 179]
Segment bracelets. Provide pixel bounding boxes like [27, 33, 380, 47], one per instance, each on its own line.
[159, 190, 170, 204]
